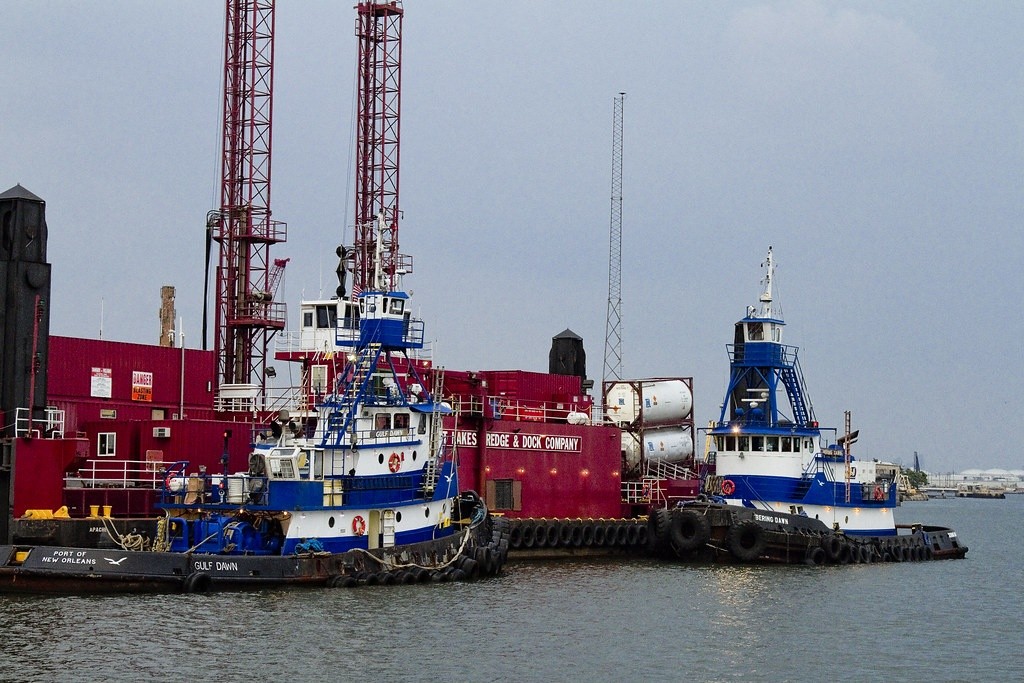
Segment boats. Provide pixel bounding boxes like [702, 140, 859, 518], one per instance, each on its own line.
[0, 206, 512, 596]
[645, 244, 970, 565]
[953, 483, 1006, 500]
[2, 181, 710, 559]
[891, 473, 929, 500]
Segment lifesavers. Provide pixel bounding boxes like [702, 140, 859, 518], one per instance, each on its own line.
[874, 486, 885, 501]
[388, 453, 401, 472]
[164, 472, 178, 492]
[725, 520, 767, 561]
[642, 485, 650, 497]
[182, 571, 213, 594]
[810, 537, 932, 566]
[328, 518, 511, 588]
[647, 508, 710, 553]
[352, 515, 366, 536]
[497, 400, 506, 414]
[721, 480, 736, 495]
[510, 524, 648, 547]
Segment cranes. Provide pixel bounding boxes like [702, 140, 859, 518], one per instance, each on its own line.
[216, 0, 291, 416]
[339, 1, 409, 299]
